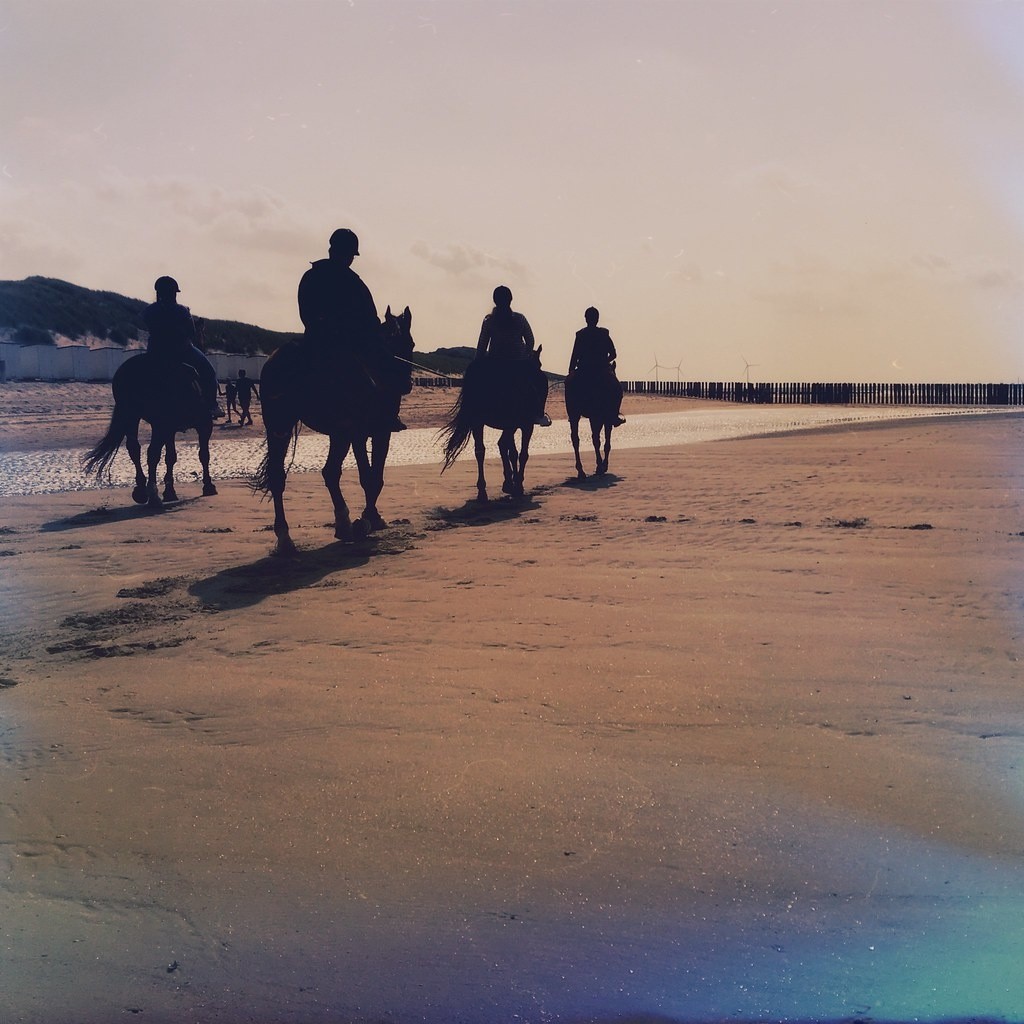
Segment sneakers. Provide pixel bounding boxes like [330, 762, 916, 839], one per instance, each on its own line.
[209, 407, 226, 417]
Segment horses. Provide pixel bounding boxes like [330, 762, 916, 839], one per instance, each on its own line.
[77, 308, 221, 515]
[429, 343, 549, 506]
[241, 304, 416, 568]
[563, 360, 624, 483]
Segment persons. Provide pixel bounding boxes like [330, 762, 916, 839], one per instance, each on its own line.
[138, 276, 226, 418]
[218, 369, 259, 426]
[476, 286, 551, 426]
[297, 229, 408, 433]
[568, 307, 626, 427]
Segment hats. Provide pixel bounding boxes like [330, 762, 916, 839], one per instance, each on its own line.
[585, 307, 599, 318]
[155, 276, 180, 292]
[329, 228, 360, 256]
[493, 285, 513, 302]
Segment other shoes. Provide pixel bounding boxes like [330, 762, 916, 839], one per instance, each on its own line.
[382, 416, 407, 432]
[613, 417, 626, 427]
[226, 419, 231, 423]
[534, 416, 550, 426]
[238, 420, 244, 426]
[245, 420, 253, 425]
